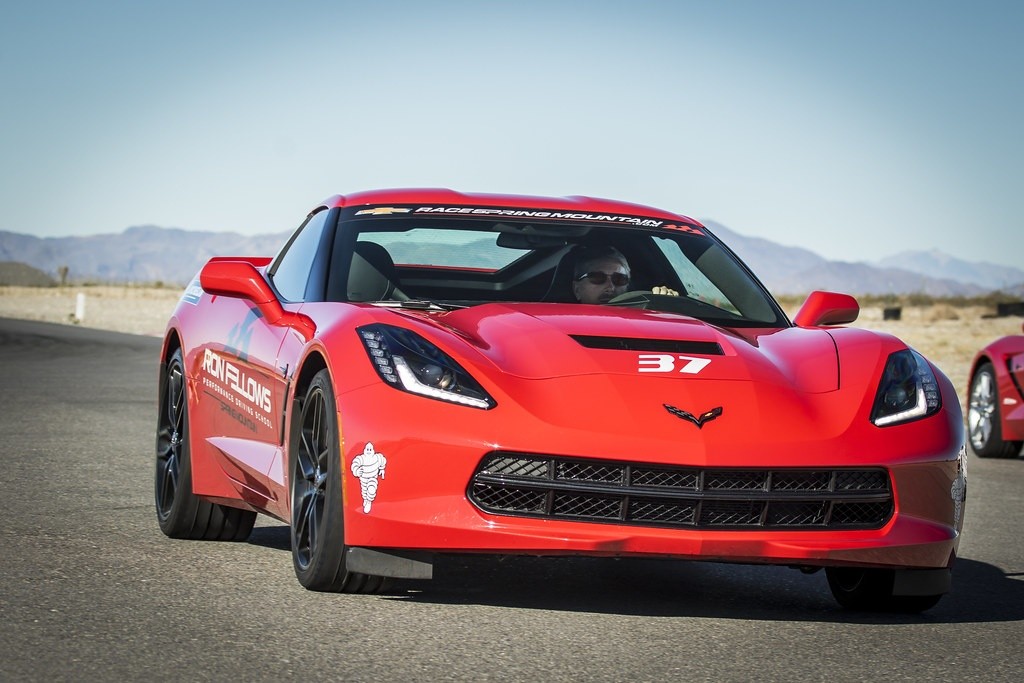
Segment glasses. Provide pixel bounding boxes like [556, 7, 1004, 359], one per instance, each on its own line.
[579, 271, 631, 286]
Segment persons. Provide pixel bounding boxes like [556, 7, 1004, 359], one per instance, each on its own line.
[572, 245, 681, 305]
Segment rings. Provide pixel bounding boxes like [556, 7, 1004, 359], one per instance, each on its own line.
[667, 289, 674, 295]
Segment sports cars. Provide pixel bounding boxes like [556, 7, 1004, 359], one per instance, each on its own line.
[966, 322, 1024, 458]
[155, 187, 969, 615]
